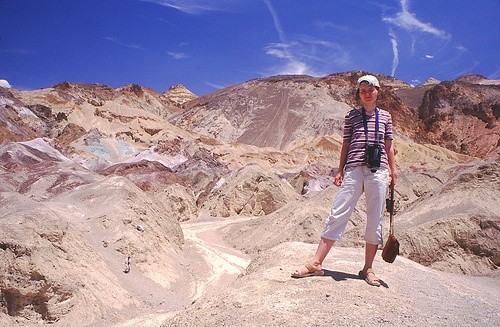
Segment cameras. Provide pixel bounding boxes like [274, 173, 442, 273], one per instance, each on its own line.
[364, 145, 381, 173]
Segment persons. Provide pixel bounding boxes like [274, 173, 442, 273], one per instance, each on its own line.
[291, 75, 397, 286]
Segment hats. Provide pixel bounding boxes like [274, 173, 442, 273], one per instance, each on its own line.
[357, 75, 380, 89]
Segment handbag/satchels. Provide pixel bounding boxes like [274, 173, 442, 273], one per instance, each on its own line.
[382, 234, 400, 263]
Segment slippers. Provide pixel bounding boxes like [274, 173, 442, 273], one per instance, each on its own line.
[359, 268, 381, 286]
[291, 263, 324, 277]
